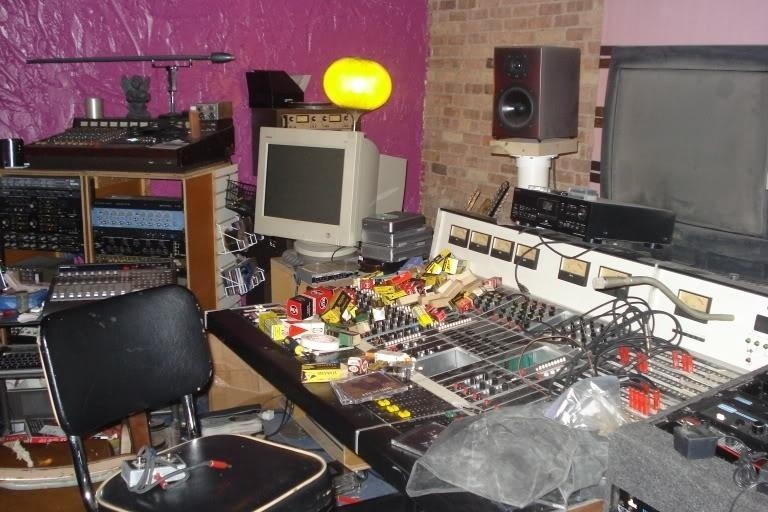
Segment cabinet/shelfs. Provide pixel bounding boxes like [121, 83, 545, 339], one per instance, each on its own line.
[0, 160, 242, 335]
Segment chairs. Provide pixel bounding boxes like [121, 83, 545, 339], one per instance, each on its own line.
[35, 283, 334, 511]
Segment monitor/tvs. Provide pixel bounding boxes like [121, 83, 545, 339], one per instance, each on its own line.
[254, 123, 408, 258]
[600, 49, 768, 299]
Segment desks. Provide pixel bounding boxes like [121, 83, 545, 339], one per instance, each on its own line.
[267, 254, 365, 305]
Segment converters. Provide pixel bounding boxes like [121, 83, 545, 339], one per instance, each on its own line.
[673, 425, 718, 458]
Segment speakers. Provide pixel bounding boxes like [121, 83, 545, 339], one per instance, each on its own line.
[492, 43, 579, 141]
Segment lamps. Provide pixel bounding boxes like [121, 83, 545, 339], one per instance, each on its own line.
[322, 56, 394, 134]
